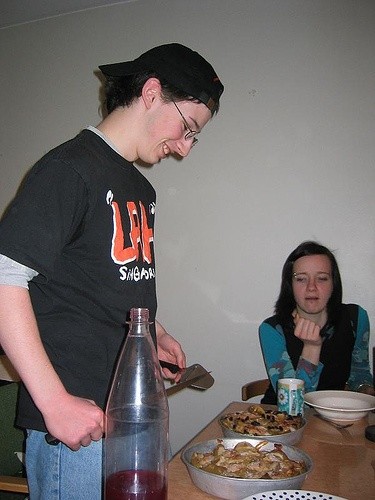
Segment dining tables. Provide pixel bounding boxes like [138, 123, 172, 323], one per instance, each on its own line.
[166, 402, 375, 500]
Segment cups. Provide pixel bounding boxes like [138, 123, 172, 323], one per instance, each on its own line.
[277, 378, 305, 416]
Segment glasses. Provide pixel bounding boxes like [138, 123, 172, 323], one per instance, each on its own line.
[165, 89, 199, 148]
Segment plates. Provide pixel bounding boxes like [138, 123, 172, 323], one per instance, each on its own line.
[242, 490, 348, 500]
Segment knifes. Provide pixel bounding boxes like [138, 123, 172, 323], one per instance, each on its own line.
[45, 371, 212, 446]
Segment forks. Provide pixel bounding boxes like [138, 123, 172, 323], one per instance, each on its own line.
[313, 414, 353, 429]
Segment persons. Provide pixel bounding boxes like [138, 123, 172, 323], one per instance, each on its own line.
[259, 241, 375, 415]
[0, 43, 224, 500]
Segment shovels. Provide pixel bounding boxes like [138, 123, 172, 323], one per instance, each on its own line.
[159, 360, 214, 389]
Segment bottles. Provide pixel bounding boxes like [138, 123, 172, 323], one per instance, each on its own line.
[104, 308, 169, 500]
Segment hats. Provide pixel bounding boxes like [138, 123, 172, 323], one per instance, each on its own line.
[97, 43, 224, 117]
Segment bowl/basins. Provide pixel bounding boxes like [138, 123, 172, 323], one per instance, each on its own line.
[217, 414, 307, 446]
[181, 438, 313, 500]
[304, 390, 375, 421]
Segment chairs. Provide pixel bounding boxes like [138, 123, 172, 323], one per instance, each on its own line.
[0, 381, 29, 500]
[242, 379, 271, 401]
